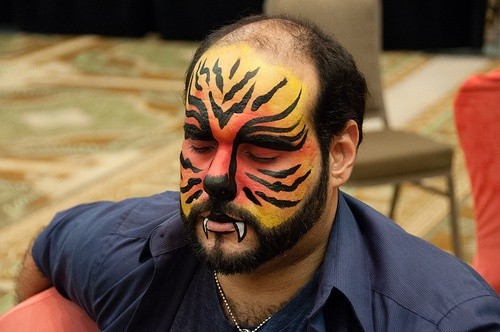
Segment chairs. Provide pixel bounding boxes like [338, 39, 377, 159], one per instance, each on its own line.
[263, 0, 462, 261]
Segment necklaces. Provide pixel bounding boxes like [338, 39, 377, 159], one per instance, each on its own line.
[213, 269, 280, 332]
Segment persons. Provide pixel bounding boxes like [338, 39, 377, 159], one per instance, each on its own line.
[16, 13, 500, 332]
